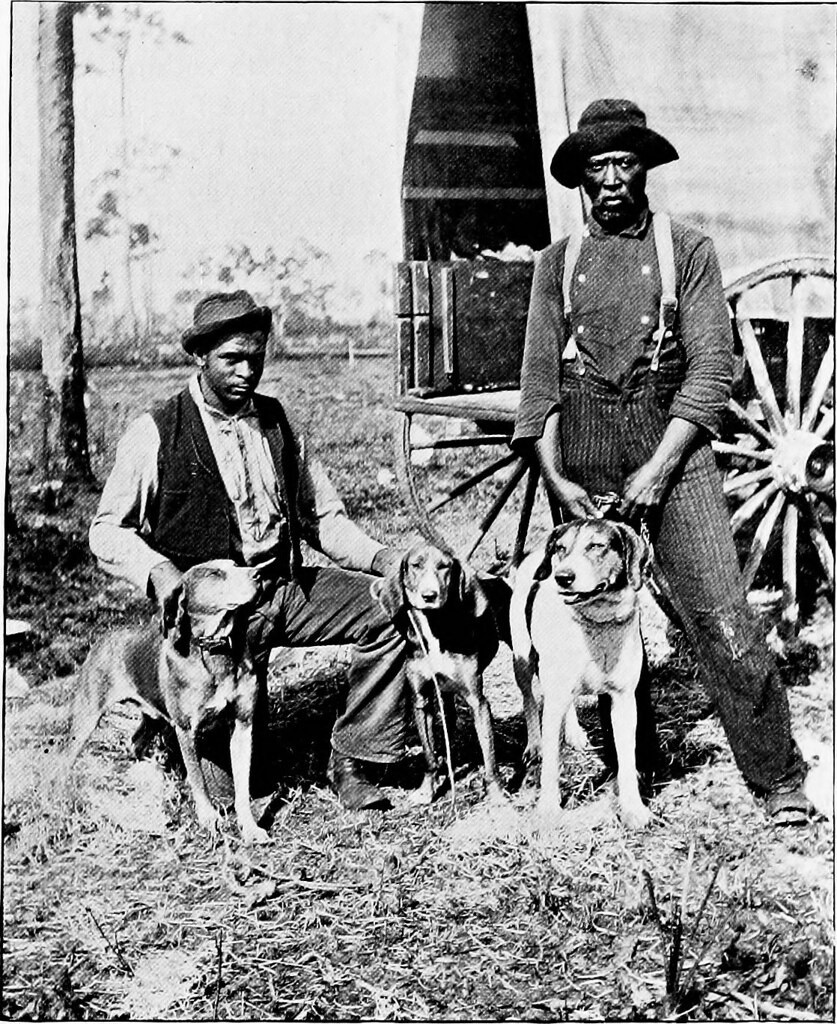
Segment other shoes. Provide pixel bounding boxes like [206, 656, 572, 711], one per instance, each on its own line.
[326, 743, 390, 810]
[759, 783, 811, 823]
[601, 767, 651, 798]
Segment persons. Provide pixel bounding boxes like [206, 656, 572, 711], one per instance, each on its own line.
[512, 100, 814, 823]
[90, 289, 455, 827]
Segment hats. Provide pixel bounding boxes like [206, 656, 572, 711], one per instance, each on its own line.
[181, 289, 272, 355]
[551, 98, 679, 189]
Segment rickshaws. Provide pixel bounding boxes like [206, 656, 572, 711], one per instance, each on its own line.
[400, 6, 835, 653]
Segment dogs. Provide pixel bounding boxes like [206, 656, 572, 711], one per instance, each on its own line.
[54, 555, 278, 847]
[503, 515, 659, 833]
[383, 542, 535, 813]
